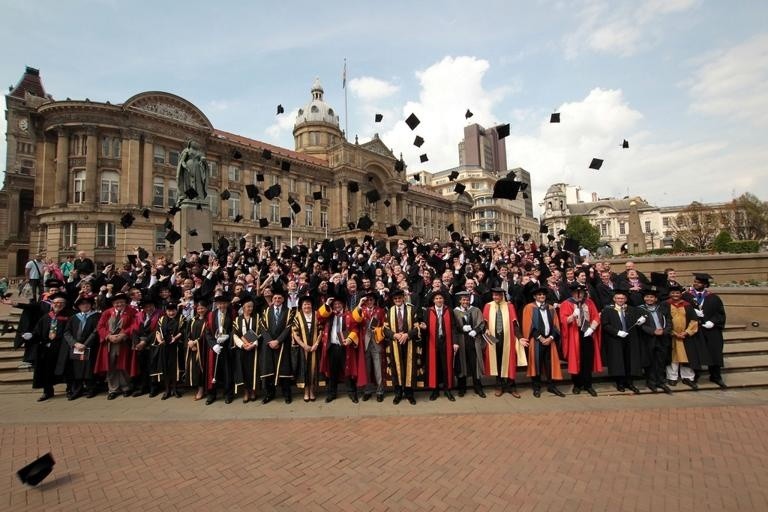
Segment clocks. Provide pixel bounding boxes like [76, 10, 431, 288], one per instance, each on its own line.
[18, 117, 29, 132]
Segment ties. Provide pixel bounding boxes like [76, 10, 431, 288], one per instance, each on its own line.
[336, 314, 342, 345]
[438, 310, 443, 337]
[397, 308, 403, 332]
[48, 313, 57, 340]
[621, 307, 626, 331]
[578, 302, 589, 333]
[496, 304, 502, 334]
[275, 308, 279, 325]
[82, 314, 86, 331]
[698, 293, 701, 299]
[115, 311, 122, 323]
[368, 309, 372, 320]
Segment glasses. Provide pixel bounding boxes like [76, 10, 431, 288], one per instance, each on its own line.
[53, 301, 64, 305]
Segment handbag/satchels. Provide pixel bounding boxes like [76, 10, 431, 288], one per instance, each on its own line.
[22, 283, 33, 300]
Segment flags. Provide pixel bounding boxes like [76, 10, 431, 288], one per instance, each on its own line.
[342, 62, 347, 89]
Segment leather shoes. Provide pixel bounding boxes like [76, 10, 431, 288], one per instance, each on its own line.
[710, 375, 727, 389]
[406, 395, 416, 405]
[310, 398, 316, 402]
[250, 394, 257, 401]
[572, 386, 581, 394]
[149, 391, 158, 398]
[66, 391, 72, 397]
[349, 394, 358, 403]
[534, 386, 540, 397]
[68, 393, 82, 400]
[458, 390, 466, 397]
[509, 391, 520, 398]
[262, 395, 274, 404]
[175, 394, 181, 399]
[669, 381, 678, 386]
[123, 390, 131, 397]
[695, 375, 701, 382]
[616, 384, 625, 392]
[225, 399, 231, 403]
[242, 398, 249, 403]
[132, 390, 142, 397]
[161, 392, 170, 400]
[304, 399, 309, 402]
[363, 393, 372, 401]
[325, 396, 336, 403]
[430, 393, 440, 401]
[445, 391, 455, 401]
[682, 378, 698, 389]
[548, 384, 562, 395]
[495, 387, 502, 396]
[37, 392, 54, 401]
[583, 386, 597, 396]
[647, 384, 657, 392]
[394, 396, 402, 405]
[108, 392, 122, 400]
[206, 400, 211, 404]
[377, 394, 383, 402]
[474, 389, 486, 398]
[285, 396, 292, 404]
[656, 384, 670, 393]
[192, 390, 204, 401]
[86, 389, 96, 398]
[625, 384, 640, 392]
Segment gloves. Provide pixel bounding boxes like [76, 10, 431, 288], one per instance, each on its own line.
[216, 334, 230, 344]
[213, 344, 224, 354]
[21, 332, 33, 341]
[702, 320, 714, 329]
[694, 309, 705, 318]
[637, 316, 647, 326]
[617, 330, 629, 338]
[463, 324, 472, 332]
[573, 308, 581, 317]
[468, 330, 477, 337]
[584, 328, 594, 337]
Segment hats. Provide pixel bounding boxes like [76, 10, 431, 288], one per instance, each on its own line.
[357, 215, 373, 230]
[238, 295, 255, 307]
[414, 173, 420, 181]
[282, 160, 291, 171]
[197, 204, 201, 210]
[401, 184, 408, 192]
[140, 294, 155, 303]
[539, 224, 548, 233]
[531, 286, 546, 292]
[163, 301, 181, 309]
[120, 211, 137, 228]
[493, 235, 500, 242]
[233, 150, 241, 158]
[189, 229, 198, 236]
[362, 289, 379, 299]
[428, 291, 447, 304]
[263, 149, 272, 159]
[466, 110, 473, 118]
[270, 288, 287, 295]
[259, 218, 269, 227]
[254, 196, 261, 204]
[692, 272, 713, 281]
[257, 174, 264, 182]
[288, 196, 294, 204]
[496, 123, 510, 140]
[291, 202, 301, 214]
[589, 158, 604, 170]
[165, 229, 181, 244]
[168, 207, 181, 215]
[138, 247, 149, 261]
[143, 209, 149, 218]
[491, 287, 505, 292]
[558, 229, 567, 236]
[281, 217, 291, 228]
[221, 189, 231, 200]
[454, 182, 466, 194]
[420, 155, 428, 162]
[331, 295, 346, 303]
[270, 184, 281, 196]
[611, 286, 629, 293]
[414, 136, 424, 147]
[127, 255, 138, 263]
[348, 222, 355, 230]
[49, 292, 66, 300]
[447, 171, 459, 181]
[211, 295, 230, 303]
[202, 243, 212, 250]
[482, 232, 490, 240]
[234, 215, 243, 222]
[567, 281, 586, 293]
[550, 113, 560, 123]
[384, 200, 391, 207]
[455, 290, 471, 295]
[669, 286, 683, 290]
[507, 171, 516, 178]
[367, 189, 381, 203]
[640, 289, 660, 296]
[245, 184, 259, 197]
[547, 235, 555, 241]
[375, 114, 383, 122]
[73, 293, 95, 307]
[165, 219, 172, 228]
[522, 233, 530, 241]
[264, 189, 276, 201]
[16, 453, 55, 486]
[45, 279, 63, 288]
[447, 224, 454, 232]
[521, 183, 528, 190]
[185, 187, 198, 199]
[391, 289, 404, 294]
[107, 291, 132, 304]
[405, 113, 420, 130]
[395, 160, 403, 172]
[624, 139, 628, 147]
[348, 180, 359, 193]
[277, 104, 284, 114]
[399, 218, 411, 231]
[492, 178, 521, 200]
[523, 192, 528, 198]
[387, 225, 398, 237]
[313, 191, 322, 200]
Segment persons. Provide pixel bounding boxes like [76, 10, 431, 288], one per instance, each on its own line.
[2, 234, 726, 405]
[175, 135, 210, 200]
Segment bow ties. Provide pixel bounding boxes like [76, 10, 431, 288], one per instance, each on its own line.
[540, 304, 549, 310]
